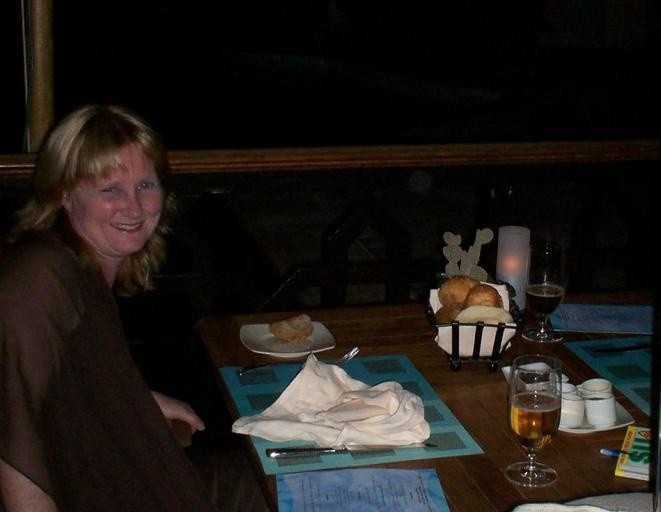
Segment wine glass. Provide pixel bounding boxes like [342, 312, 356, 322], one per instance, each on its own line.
[521, 242, 565, 344]
[503, 353, 562, 489]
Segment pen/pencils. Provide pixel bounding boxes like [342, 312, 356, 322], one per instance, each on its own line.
[600, 449, 620, 458]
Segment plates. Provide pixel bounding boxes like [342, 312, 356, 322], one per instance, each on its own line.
[240, 318, 337, 358]
[558, 399, 636, 433]
[502, 361, 570, 391]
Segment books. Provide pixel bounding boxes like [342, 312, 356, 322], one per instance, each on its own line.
[615, 426, 651, 482]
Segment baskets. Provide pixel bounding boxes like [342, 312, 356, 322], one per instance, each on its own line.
[424, 278, 523, 372]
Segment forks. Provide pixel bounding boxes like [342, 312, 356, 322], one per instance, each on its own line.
[237, 344, 361, 379]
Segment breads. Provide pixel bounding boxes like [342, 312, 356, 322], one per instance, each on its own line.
[271, 314, 313, 340]
[435, 277, 502, 323]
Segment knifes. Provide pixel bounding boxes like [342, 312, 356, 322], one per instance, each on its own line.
[265, 441, 440, 459]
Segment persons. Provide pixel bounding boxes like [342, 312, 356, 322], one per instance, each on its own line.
[0, 104, 216, 512]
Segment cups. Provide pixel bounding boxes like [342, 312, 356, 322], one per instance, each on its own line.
[576, 376, 614, 400]
[583, 392, 617, 429]
[556, 381, 575, 392]
[557, 392, 586, 429]
